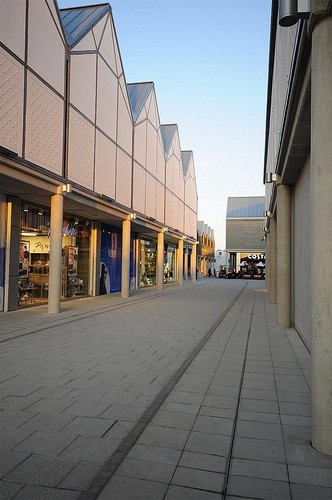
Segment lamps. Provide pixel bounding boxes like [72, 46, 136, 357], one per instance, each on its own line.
[63, 183, 72, 193]
[183, 234, 187, 239]
[265, 172, 282, 185]
[262, 210, 271, 217]
[131, 213, 137, 220]
[261, 235, 265, 241]
[278, 0, 310, 28]
[163, 226, 169, 232]
[262, 227, 268, 232]
[196, 240, 200, 245]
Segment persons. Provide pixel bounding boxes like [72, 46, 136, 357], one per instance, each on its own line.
[24, 246, 29, 261]
[100, 264, 108, 295]
[74, 250, 78, 265]
